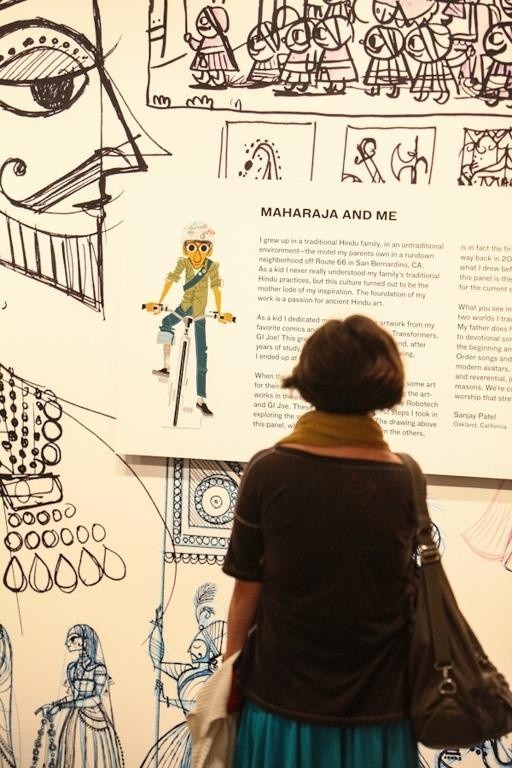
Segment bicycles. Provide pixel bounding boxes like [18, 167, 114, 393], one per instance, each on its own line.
[142, 303, 236, 427]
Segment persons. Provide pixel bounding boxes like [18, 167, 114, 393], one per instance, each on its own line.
[220, 316, 431, 766]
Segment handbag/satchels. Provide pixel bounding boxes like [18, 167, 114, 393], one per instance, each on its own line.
[394, 453, 512, 750]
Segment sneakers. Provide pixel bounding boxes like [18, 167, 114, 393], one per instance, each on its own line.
[151, 366, 170, 379]
[196, 400, 214, 418]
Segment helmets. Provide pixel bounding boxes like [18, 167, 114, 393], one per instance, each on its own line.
[180, 221, 216, 257]
